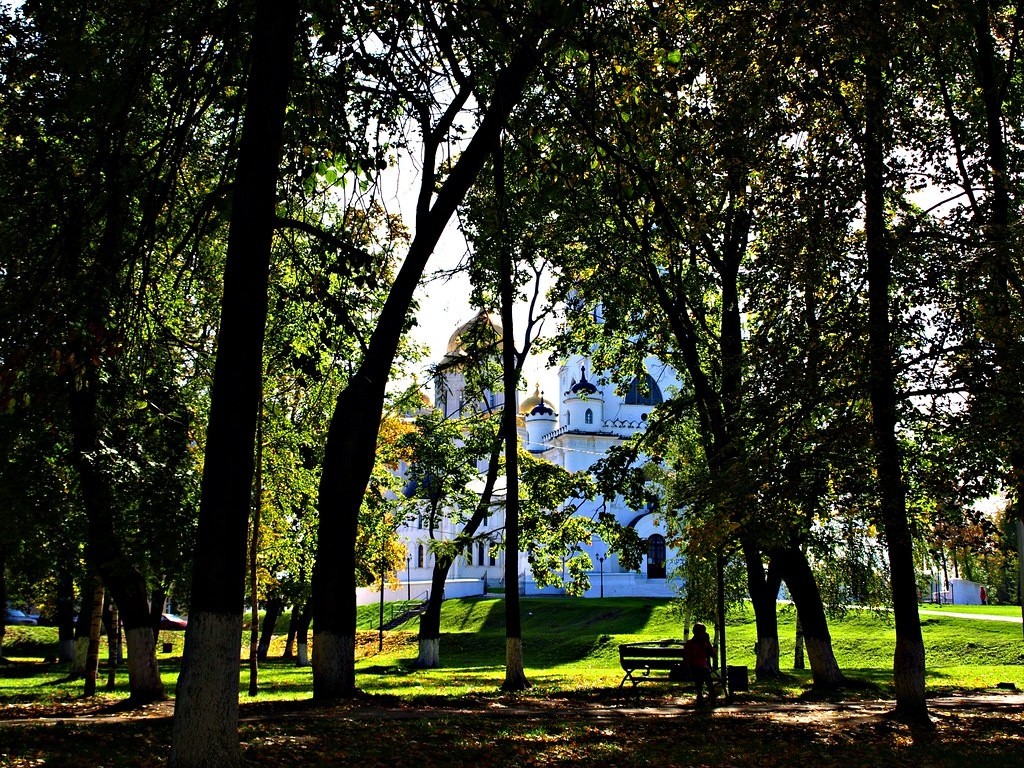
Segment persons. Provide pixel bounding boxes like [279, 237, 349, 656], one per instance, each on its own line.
[682, 623, 717, 705]
[980, 586, 986, 604]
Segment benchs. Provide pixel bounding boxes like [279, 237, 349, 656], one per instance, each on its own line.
[619, 642, 728, 698]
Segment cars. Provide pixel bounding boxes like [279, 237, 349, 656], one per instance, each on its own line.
[159, 613, 187, 630]
[5, 609, 38, 627]
